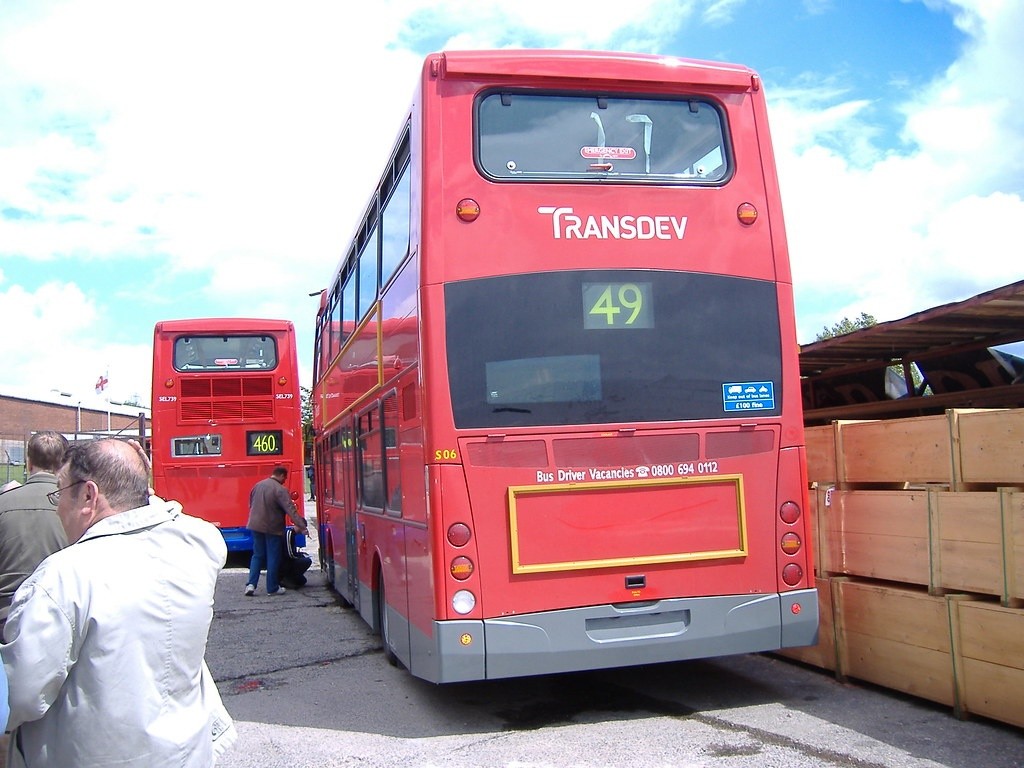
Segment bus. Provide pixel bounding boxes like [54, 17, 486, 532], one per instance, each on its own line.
[148, 316, 305, 556]
[309, 46, 822, 685]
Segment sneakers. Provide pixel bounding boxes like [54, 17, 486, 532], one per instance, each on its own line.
[245, 584, 255, 595]
[268, 585, 286, 596]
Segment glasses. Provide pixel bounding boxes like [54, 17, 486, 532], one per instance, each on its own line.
[46, 480, 89, 506]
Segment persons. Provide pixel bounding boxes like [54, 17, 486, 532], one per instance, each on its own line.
[279, 518, 312, 590]
[244, 467, 309, 596]
[305, 465, 317, 501]
[0, 428, 237, 768]
